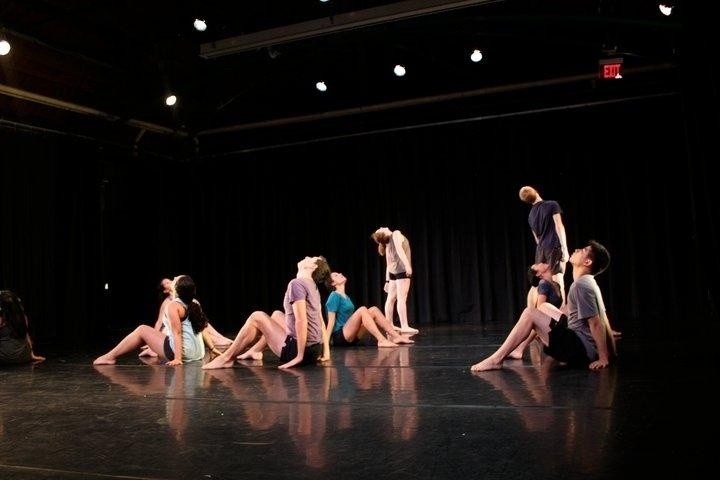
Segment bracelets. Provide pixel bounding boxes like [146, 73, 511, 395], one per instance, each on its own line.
[385, 280, 389, 283]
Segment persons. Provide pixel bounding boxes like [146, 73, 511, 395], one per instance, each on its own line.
[0, 289, 47, 365]
[94, 275, 234, 371]
[202, 256, 331, 370]
[371, 228, 418, 332]
[94, 344, 418, 476]
[325, 272, 415, 346]
[473, 370, 618, 480]
[470, 185, 622, 372]
